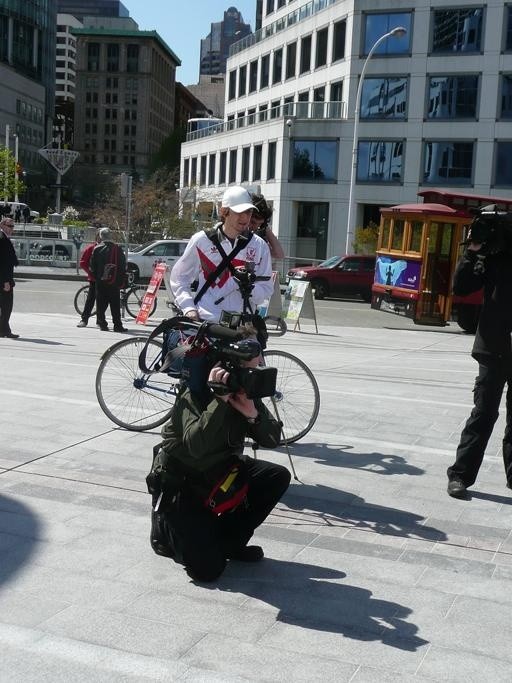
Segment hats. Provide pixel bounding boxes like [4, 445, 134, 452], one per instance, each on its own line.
[221, 186, 260, 214]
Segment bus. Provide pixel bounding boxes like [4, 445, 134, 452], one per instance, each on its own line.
[368, 188, 512, 328]
[0, 200, 40, 224]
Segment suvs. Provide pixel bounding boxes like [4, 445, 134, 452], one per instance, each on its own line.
[150, 220, 161, 232]
[287, 254, 376, 302]
[121, 238, 200, 288]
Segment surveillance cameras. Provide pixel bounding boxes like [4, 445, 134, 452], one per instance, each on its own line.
[286, 119, 293, 127]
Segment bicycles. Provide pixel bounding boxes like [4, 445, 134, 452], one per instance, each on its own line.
[74, 273, 160, 320]
[95, 310, 321, 447]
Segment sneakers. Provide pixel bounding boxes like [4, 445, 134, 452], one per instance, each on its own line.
[151, 510, 174, 556]
[226, 544, 263, 563]
[114, 326, 128, 331]
[100, 326, 109, 331]
[76, 322, 86, 326]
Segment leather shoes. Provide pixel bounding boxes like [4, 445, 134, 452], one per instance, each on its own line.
[447, 480, 466, 498]
[7, 333, 19, 338]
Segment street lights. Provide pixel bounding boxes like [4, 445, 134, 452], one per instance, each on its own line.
[12, 132, 21, 204]
[345, 26, 409, 256]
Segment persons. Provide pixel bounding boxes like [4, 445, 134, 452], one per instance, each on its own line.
[15, 206, 21, 223]
[170, 186, 274, 323]
[0, 204, 14, 222]
[90, 227, 128, 331]
[147, 329, 291, 582]
[446, 204, 512, 499]
[22, 205, 30, 223]
[0, 217, 19, 338]
[77, 230, 102, 328]
[250, 193, 284, 258]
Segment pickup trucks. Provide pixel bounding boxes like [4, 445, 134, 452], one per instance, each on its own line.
[89, 209, 127, 229]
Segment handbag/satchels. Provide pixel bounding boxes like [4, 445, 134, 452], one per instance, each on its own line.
[161, 328, 224, 378]
[103, 264, 120, 280]
